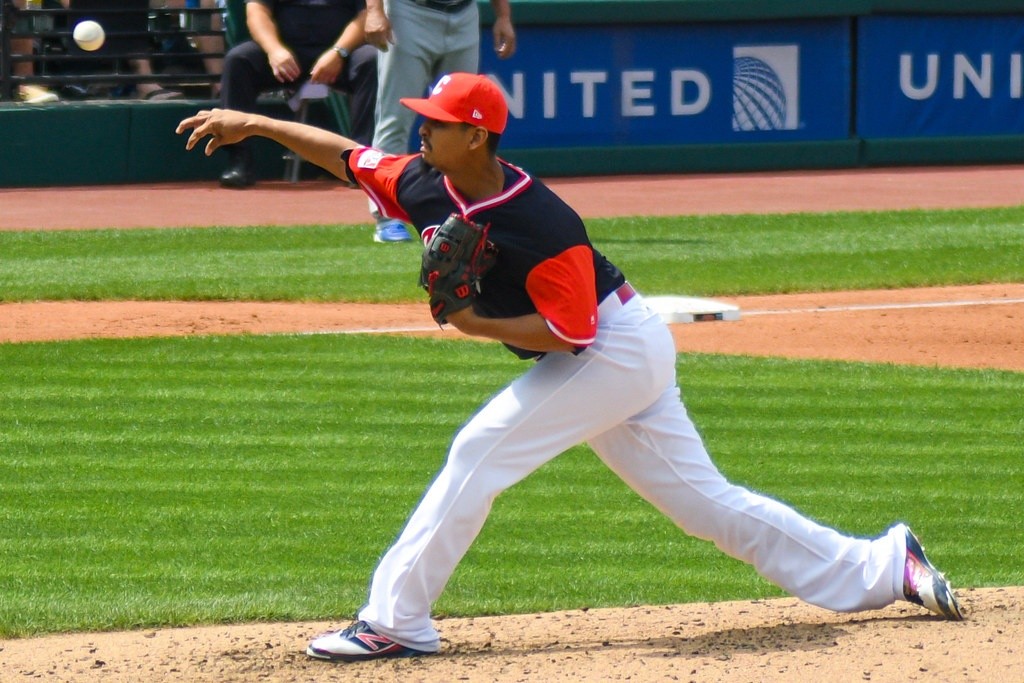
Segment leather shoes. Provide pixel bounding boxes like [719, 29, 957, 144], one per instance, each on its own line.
[221, 154, 271, 186]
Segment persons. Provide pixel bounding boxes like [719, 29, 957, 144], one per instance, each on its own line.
[0, 0, 228, 102]
[176, 72, 966, 661]
[365, 0, 515, 243]
[216, 0, 379, 188]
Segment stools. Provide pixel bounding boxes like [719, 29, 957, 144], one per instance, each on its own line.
[283, 81, 354, 183]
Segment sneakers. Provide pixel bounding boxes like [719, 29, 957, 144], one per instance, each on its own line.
[902, 525, 965, 621]
[306, 619, 434, 662]
[373, 219, 412, 242]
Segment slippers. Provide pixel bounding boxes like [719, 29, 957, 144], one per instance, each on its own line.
[146, 87, 184, 100]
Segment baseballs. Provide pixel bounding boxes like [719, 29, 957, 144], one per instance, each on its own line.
[72, 19, 107, 52]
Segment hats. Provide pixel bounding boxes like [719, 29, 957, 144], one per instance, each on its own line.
[400, 72, 508, 134]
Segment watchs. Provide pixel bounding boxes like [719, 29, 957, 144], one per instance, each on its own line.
[330, 46, 350, 61]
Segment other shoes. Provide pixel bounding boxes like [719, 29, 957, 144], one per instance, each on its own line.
[17, 87, 58, 103]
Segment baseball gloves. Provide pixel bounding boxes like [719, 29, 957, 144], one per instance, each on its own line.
[415, 212, 500, 332]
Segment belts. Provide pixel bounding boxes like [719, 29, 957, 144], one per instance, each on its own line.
[412, 0, 474, 13]
[616, 284, 635, 307]
